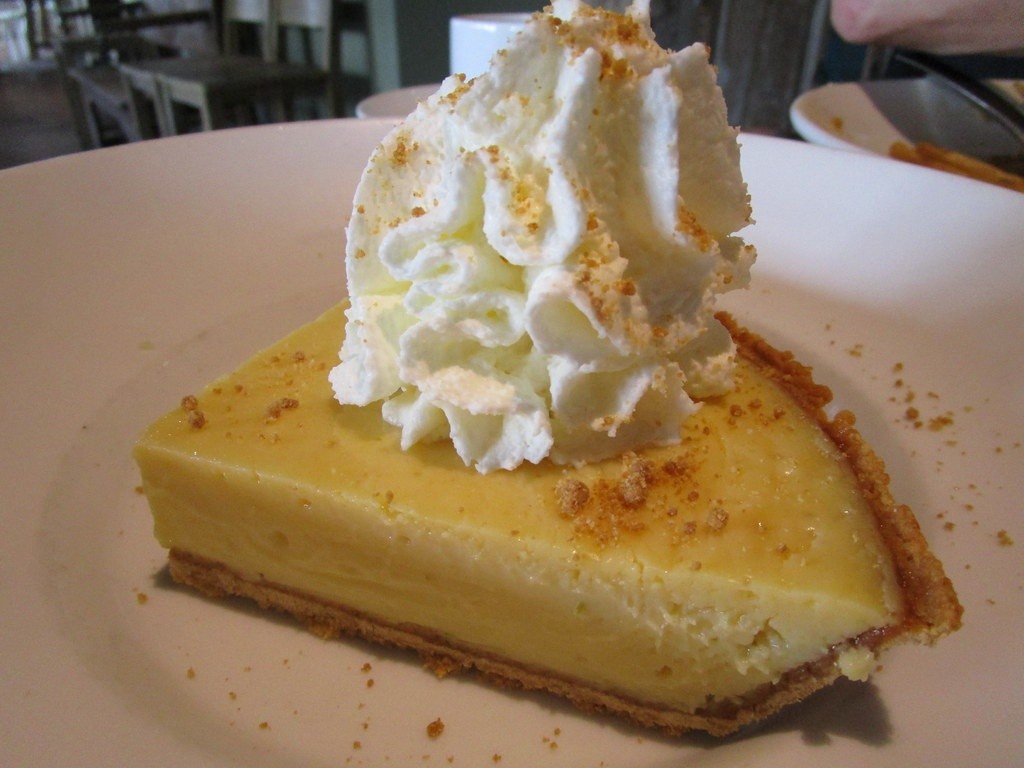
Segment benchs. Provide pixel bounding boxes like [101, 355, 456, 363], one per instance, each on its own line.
[67, 0, 225, 143]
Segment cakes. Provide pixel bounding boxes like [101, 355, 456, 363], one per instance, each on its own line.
[129, 2, 967, 735]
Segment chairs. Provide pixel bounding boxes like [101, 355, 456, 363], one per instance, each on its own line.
[115, 0, 274, 141]
[151, 0, 343, 135]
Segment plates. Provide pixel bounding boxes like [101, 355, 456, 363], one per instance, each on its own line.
[357, 85, 441, 119]
[790, 78, 1024, 194]
[0, 119, 1024, 767]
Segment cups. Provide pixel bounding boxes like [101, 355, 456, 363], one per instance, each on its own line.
[449, 11, 535, 80]
